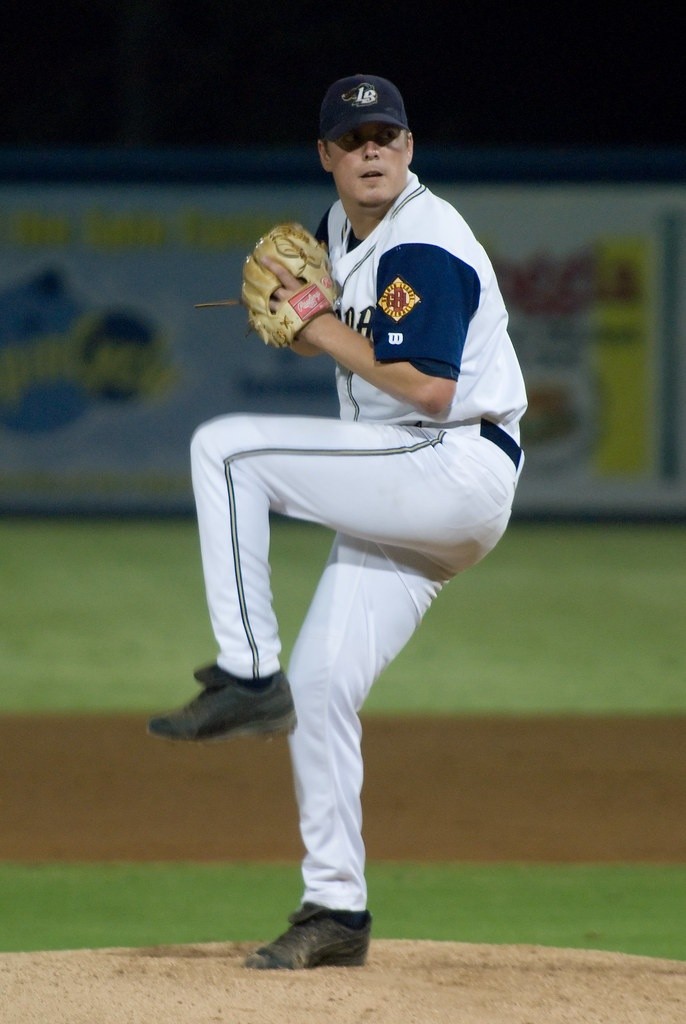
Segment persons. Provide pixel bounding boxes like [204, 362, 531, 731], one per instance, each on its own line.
[149, 75, 525, 969]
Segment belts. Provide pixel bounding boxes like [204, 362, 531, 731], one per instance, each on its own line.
[478, 417, 523, 472]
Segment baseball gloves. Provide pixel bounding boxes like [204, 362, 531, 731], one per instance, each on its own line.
[239, 222, 338, 346]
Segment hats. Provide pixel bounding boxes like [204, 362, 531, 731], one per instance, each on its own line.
[318, 72, 412, 146]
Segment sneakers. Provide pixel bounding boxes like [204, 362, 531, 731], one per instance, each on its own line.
[244, 901, 376, 968]
[144, 665, 299, 744]
